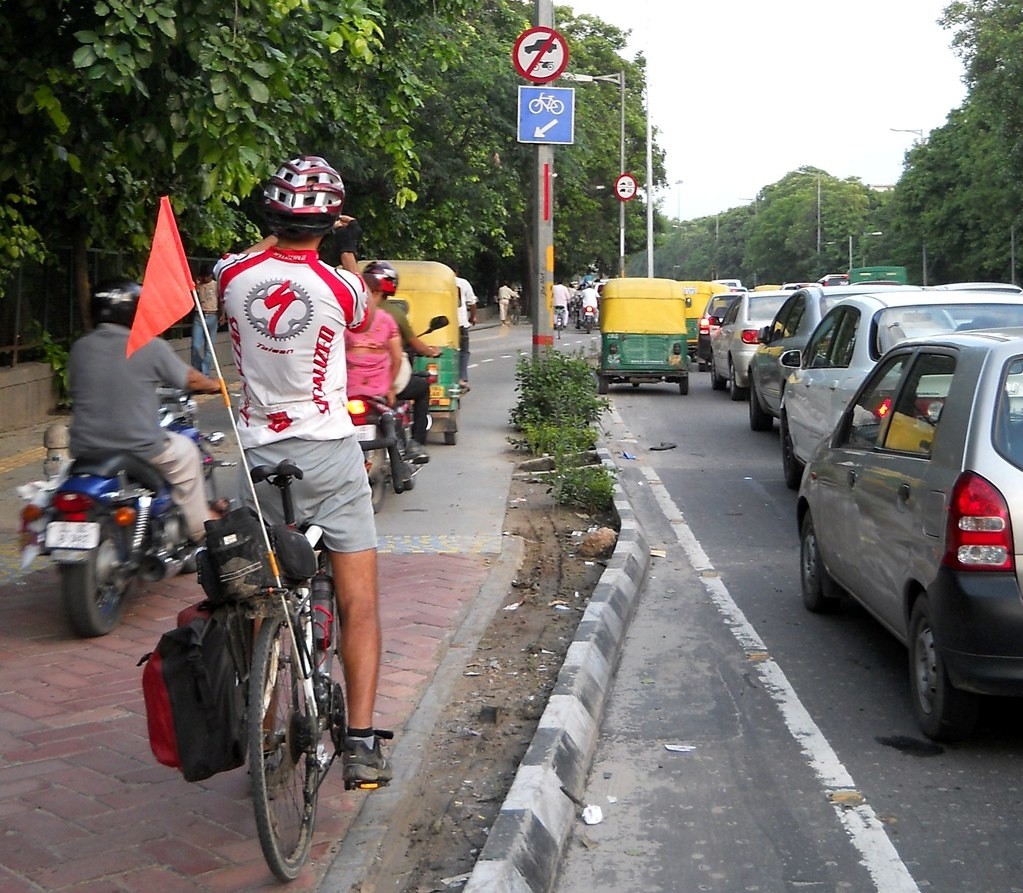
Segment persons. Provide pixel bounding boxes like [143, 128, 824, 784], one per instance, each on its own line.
[494, 280, 601, 330]
[362, 260, 443, 464]
[344, 273, 416, 494]
[445, 261, 480, 391]
[213, 156, 394, 789]
[66, 276, 226, 548]
[191, 264, 226, 379]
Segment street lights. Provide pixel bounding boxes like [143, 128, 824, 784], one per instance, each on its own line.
[890, 128, 925, 284]
[794, 171, 821, 253]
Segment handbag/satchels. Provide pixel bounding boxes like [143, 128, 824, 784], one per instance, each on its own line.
[137, 619, 248, 784]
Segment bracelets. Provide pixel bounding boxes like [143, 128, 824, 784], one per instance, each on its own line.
[427, 346, 434, 357]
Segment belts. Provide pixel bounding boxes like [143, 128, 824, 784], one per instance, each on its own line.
[195, 311, 219, 315]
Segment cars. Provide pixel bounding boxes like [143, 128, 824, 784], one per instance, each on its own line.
[780, 290, 1023, 489]
[708, 290, 806, 401]
[924, 282, 1023, 329]
[748, 286, 927, 433]
[754, 274, 850, 291]
[697, 294, 740, 372]
[795, 327, 1023, 742]
[712, 280, 748, 292]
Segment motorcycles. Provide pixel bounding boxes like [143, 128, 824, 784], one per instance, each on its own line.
[350, 316, 449, 515]
[597, 278, 692, 396]
[20, 387, 242, 638]
[338, 260, 460, 446]
[679, 282, 731, 362]
[583, 306, 598, 334]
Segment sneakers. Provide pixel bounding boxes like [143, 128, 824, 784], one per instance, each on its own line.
[342, 735, 394, 783]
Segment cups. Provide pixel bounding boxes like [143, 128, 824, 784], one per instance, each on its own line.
[310, 575, 332, 639]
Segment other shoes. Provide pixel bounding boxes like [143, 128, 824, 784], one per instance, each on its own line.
[407, 439, 429, 458]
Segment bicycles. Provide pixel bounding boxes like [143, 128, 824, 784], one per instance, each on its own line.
[555, 306, 565, 339]
[249, 456, 388, 882]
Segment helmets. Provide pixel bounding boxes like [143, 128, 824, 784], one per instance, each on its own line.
[360, 259, 398, 295]
[258, 155, 345, 241]
[584, 281, 593, 288]
[90, 280, 143, 330]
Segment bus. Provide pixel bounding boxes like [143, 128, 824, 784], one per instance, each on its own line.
[848, 267, 908, 286]
